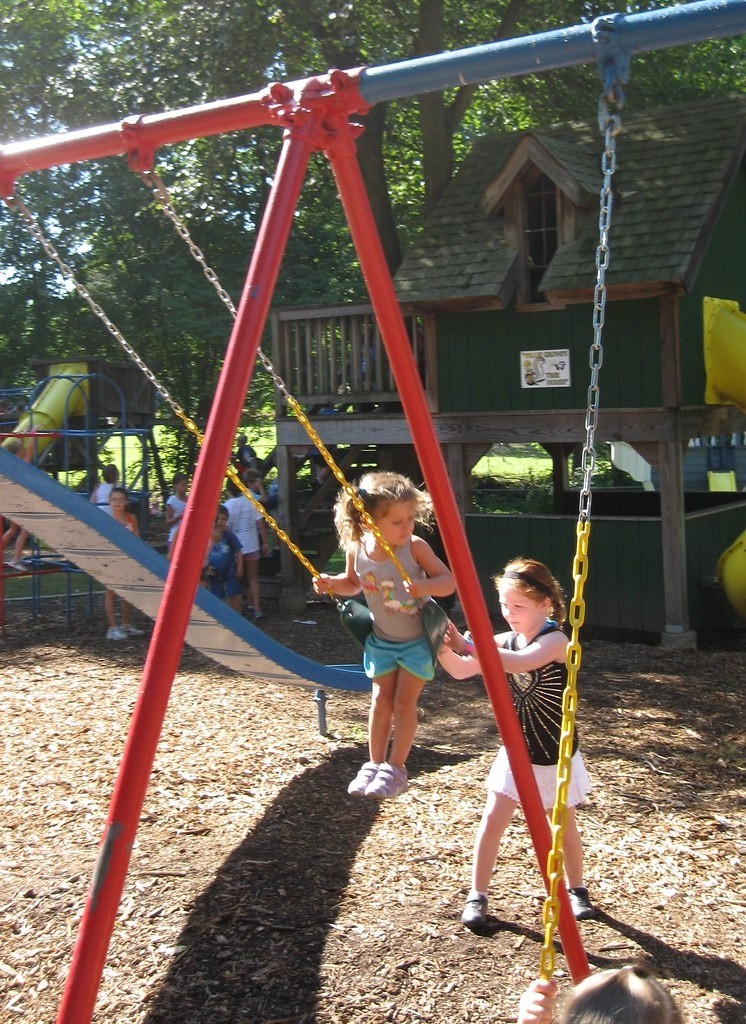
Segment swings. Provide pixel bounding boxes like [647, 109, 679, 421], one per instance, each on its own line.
[28, 222, 452, 666]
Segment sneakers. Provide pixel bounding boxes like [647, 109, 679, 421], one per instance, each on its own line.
[567, 887, 594, 921]
[460, 891, 490, 937]
[105, 627, 129, 642]
[346, 760, 409, 802]
[119, 626, 146, 637]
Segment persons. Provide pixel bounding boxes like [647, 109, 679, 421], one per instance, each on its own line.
[90, 464, 119, 516]
[312, 471, 456, 802]
[0, 424, 42, 572]
[436, 557, 597, 929]
[306, 398, 359, 493]
[103, 487, 146, 640]
[166, 435, 270, 618]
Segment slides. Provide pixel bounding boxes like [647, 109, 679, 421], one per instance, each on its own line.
[1, 365, 89, 465]
[0, 447, 373, 692]
[704, 297, 746, 616]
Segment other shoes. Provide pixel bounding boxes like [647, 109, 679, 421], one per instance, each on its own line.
[255, 608, 264, 619]
[7, 559, 28, 573]
[309, 477, 323, 492]
[246, 605, 256, 614]
